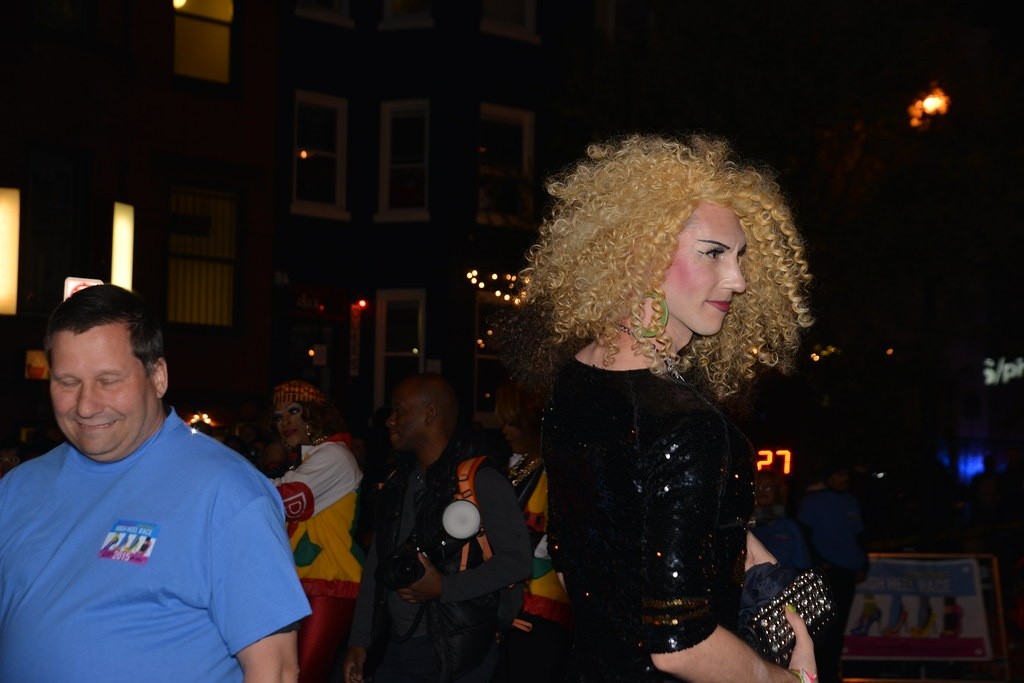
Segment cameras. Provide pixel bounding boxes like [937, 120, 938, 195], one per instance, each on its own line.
[381, 533, 429, 591]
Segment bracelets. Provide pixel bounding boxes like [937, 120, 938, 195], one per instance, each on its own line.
[789, 667, 817, 683]
[824, 478, 831, 488]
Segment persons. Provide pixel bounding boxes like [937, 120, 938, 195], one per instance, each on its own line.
[0, 284, 313, 682]
[188, 378, 394, 682]
[750, 449, 872, 683]
[491, 379, 574, 683]
[343, 373, 533, 683]
[0, 411, 65, 479]
[483, 133, 822, 683]
[859, 448, 1023, 635]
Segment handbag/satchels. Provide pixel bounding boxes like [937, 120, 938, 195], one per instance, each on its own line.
[752, 569, 834, 657]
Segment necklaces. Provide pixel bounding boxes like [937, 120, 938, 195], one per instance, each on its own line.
[616, 323, 685, 381]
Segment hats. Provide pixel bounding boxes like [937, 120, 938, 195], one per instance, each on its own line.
[273, 380, 324, 402]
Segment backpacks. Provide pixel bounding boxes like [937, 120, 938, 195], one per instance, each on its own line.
[457, 455, 532, 632]
